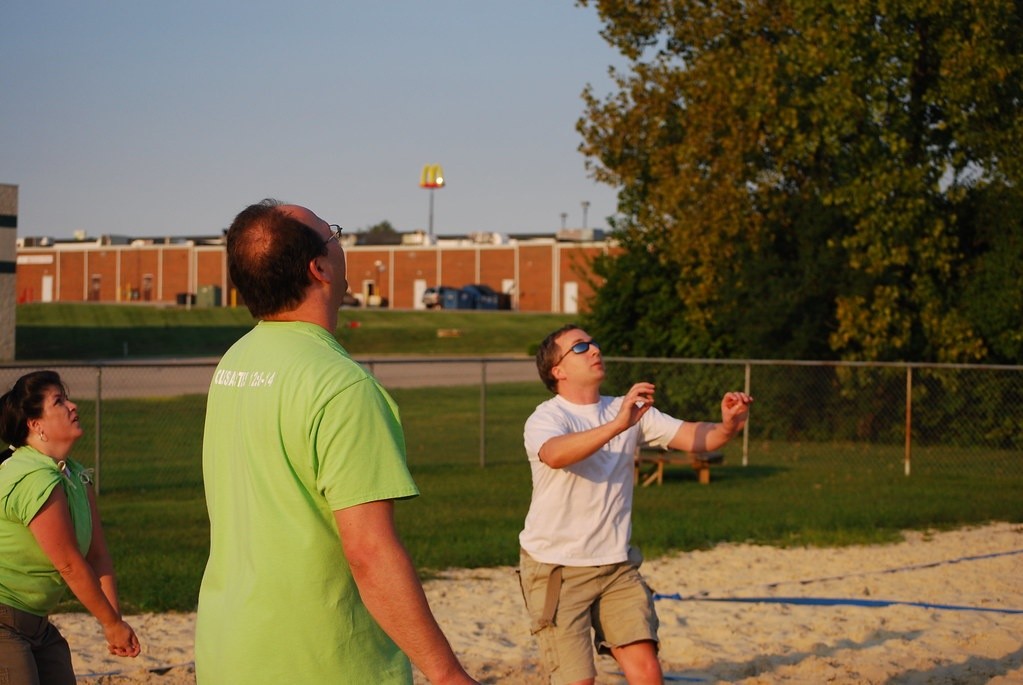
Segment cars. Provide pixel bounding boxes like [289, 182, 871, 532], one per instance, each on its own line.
[422, 287, 442, 309]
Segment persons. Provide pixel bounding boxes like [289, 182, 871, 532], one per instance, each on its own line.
[0, 372, 141, 685]
[516, 324, 754, 685]
[194, 196, 480, 685]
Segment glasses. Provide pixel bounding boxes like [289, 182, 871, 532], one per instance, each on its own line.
[309, 224, 343, 261]
[554, 339, 601, 367]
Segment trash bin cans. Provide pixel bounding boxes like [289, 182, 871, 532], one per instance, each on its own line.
[196, 284, 220, 306]
[444, 288, 473, 309]
[464, 285, 511, 309]
